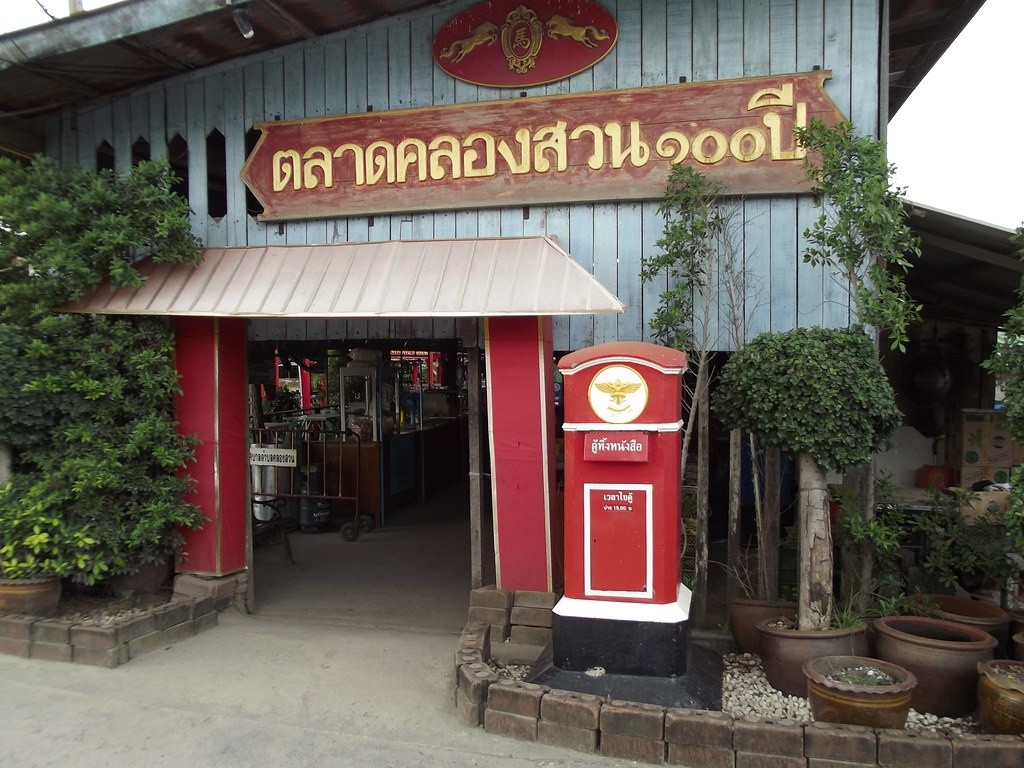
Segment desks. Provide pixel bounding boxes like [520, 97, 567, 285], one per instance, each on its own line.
[283, 414, 340, 441]
[828, 484, 960, 549]
[264, 423, 290, 444]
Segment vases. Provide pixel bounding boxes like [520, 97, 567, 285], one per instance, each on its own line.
[969, 544, 1024, 632]
[1012, 632, 1024, 660]
[908, 594, 1011, 659]
[873, 616, 999, 718]
[975, 660, 1024, 735]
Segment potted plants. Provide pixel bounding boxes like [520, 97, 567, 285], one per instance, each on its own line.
[803, 655, 918, 729]
[727, 534, 798, 655]
[0, 481, 108, 617]
[712, 324, 904, 699]
[112, 473, 212, 599]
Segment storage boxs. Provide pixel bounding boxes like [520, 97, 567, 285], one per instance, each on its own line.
[948, 407, 1024, 488]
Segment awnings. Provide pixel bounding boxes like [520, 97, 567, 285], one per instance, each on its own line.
[54, 235, 630, 318]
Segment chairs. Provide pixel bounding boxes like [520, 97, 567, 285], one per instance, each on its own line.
[251, 496, 297, 561]
